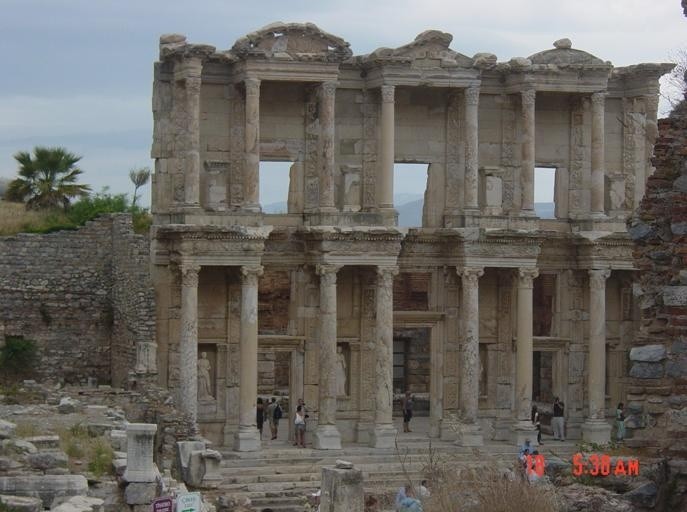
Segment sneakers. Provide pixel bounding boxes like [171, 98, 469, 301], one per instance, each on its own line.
[271, 436, 277, 440]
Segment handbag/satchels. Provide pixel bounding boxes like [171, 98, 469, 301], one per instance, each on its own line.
[274, 407, 281, 419]
[295, 416, 305, 424]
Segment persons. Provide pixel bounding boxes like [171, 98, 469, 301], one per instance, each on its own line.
[615, 401, 632, 442]
[518, 438, 544, 481]
[198, 351, 215, 400]
[263, 397, 282, 440]
[392, 478, 432, 512]
[402, 390, 415, 434]
[292, 406, 308, 448]
[530, 404, 544, 446]
[335, 345, 348, 395]
[550, 396, 566, 442]
[257, 397, 265, 439]
[297, 399, 309, 423]
